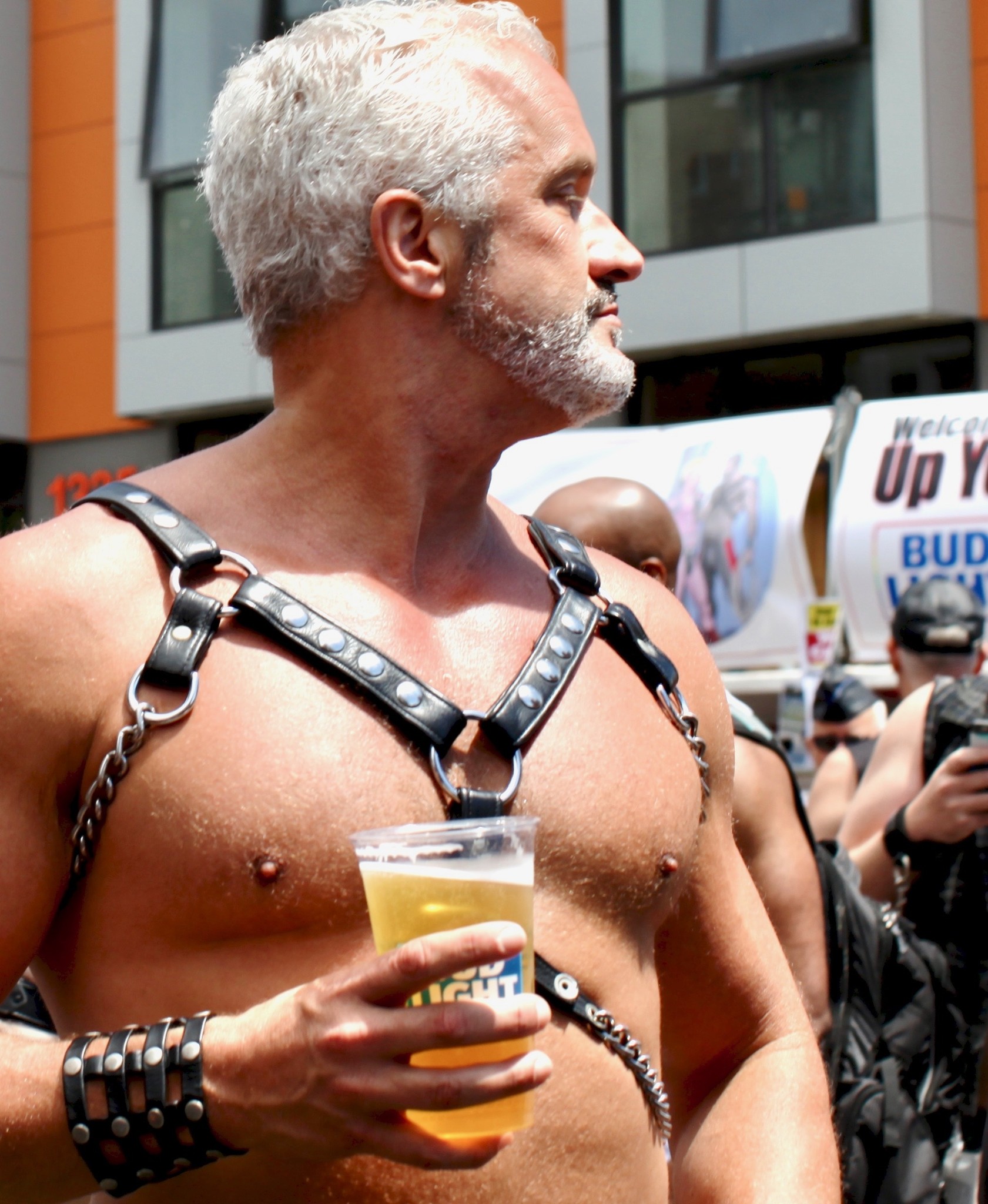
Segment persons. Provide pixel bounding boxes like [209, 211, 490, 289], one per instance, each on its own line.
[1, 0, 844, 1204]
[531, 478, 985, 1202]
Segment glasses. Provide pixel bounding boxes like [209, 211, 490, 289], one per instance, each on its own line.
[813, 734, 867, 754]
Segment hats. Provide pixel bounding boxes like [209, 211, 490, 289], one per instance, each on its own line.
[812, 664, 880, 722]
[895, 579, 984, 648]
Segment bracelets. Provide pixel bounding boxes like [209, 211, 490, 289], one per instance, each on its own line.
[61, 1011, 248, 1201]
[881, 802, 928, 871]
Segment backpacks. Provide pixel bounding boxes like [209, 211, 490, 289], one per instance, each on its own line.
[813, 832, 981, 1204]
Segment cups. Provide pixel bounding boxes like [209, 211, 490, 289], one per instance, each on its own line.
[351, 812, 535, 1138]
